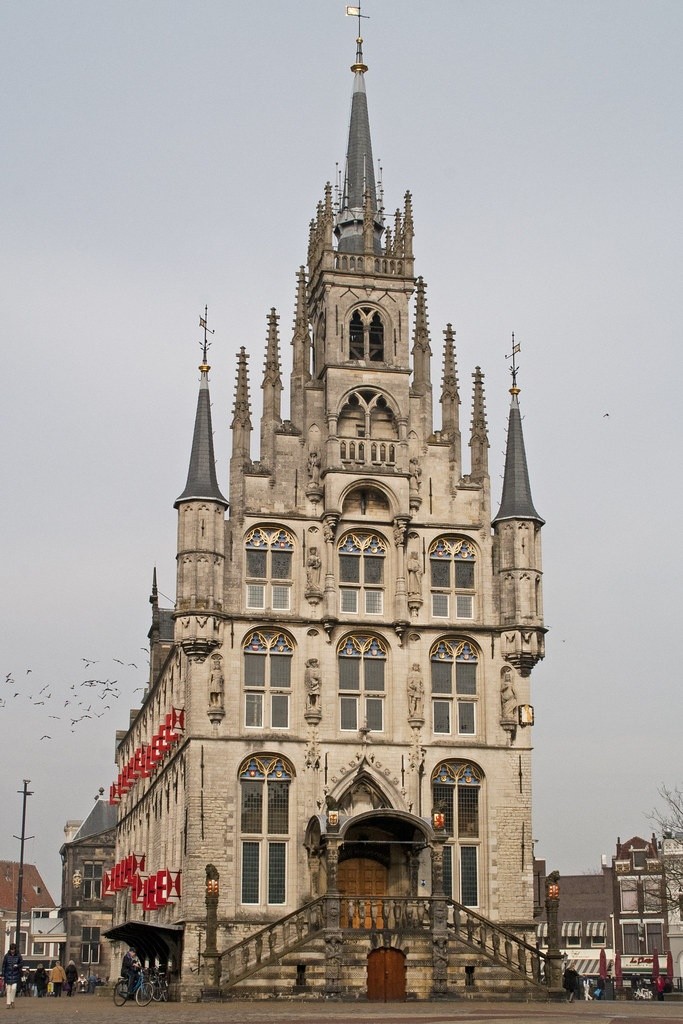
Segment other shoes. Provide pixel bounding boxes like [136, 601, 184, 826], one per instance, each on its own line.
[11, 1003, 15, 1008]
[6, 1004, 10, 1009]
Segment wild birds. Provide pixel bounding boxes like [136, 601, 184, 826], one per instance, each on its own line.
[0, 647, 151, 740]
[603, 414, 609, 418]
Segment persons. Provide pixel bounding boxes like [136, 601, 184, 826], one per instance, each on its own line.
[0, 959, 101, 998]
[655, 974, 666, 1001]
[564, 963, 605, 1003]
[121, 946, 142, 1000]
[2, 943, 24, 1009]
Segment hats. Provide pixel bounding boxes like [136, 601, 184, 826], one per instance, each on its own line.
[133, 960, 137, 962]
[130, 947, 136, 951]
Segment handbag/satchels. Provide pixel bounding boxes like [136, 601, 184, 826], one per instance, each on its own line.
[48, 982, 54, 992]
[63, 982, 71, 991]
[594, 989, 602, 996]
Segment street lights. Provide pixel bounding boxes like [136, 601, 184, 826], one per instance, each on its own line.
[12, 778, 36, 956]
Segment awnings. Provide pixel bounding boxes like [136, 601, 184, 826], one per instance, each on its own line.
[561, 959, 613, 976]
[536, 922, 606, 938]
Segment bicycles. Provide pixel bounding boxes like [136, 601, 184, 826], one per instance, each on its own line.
[143, 965, 170, 1003]
[111, 967, 154, 1007]
